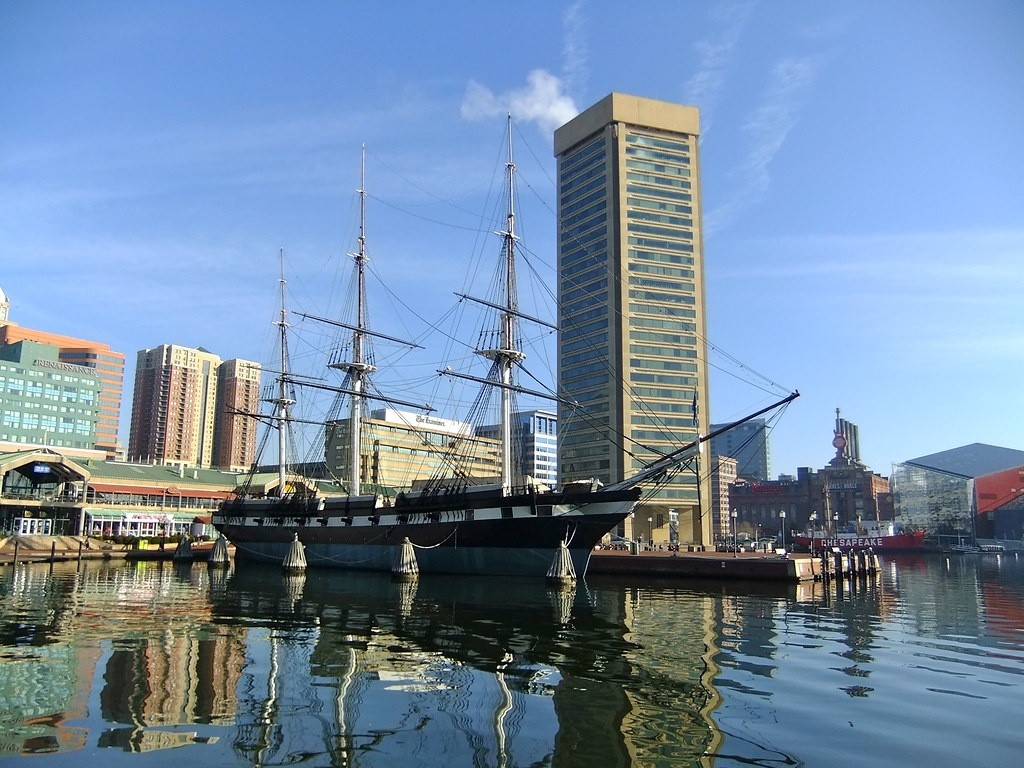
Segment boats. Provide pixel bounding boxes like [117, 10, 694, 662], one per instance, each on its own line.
[950, 538, 980, 553]
[792, 471, 926, 554]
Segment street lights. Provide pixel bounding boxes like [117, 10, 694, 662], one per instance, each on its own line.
[646, 517, 652, 548]
[730, 507, 737, 560]
[779, 510, 786, 549]
[833, 512, 838, 547]
[810, 511, 817, 552]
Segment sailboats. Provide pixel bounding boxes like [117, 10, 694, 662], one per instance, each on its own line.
[212, 111, 802, 574]
